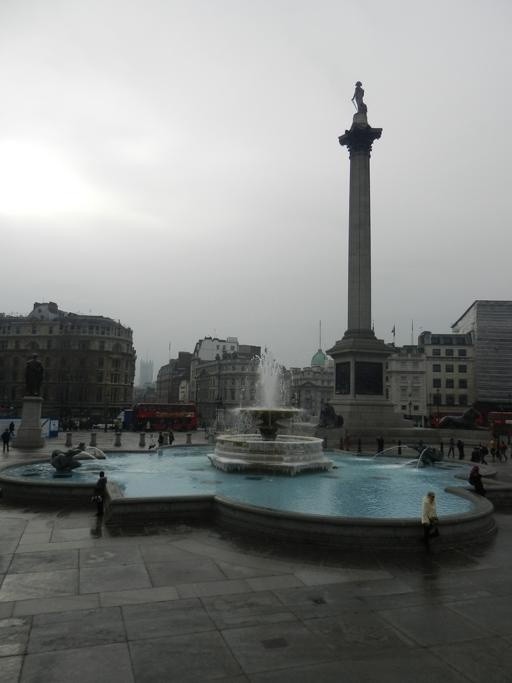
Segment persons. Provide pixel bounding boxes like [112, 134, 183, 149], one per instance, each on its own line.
[1, 429, 10, 452]
[9, 422, 14, 436]
[324, 434, 509, 464]
[148, 431, 174, 449]
[469, 465, 483, 496]
[422, 491, 440, 553]
[90, 471, 107, 537]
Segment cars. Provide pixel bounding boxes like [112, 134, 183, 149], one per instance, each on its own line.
[93, 423, 115, 430]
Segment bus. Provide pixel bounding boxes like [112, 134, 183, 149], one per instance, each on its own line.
[486, 411, 512, 428]
[134, 403, 197, 432]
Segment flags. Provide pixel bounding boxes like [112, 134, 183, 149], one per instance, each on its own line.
[391, 325, 395, 336]
